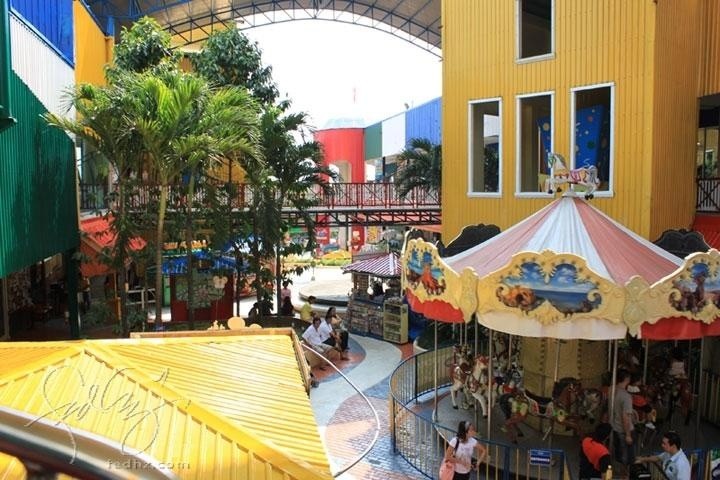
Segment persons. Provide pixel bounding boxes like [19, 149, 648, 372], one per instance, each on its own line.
[248, 302, 259, 318]
[81, 273, 92, 311]
[302, 305, 349, 373]
[636, 431, 691, 480]
[446, 420, 486, 480]
[369, 279, 383, 303]
[300, 295, 315, 322]
[607, 369, 636, 466]
[265, 296, 274, 315]
[280, 280, 292, 307]
[577, 423, 612, 480]
[281, 295, 296, 315]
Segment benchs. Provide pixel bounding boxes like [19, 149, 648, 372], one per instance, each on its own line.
[299, 339, 344, 367]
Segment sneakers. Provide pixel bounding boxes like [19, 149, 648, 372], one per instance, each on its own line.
[319, 357, 350, 370]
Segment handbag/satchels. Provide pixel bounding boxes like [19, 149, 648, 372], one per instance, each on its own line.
[439, 458, 454, 480]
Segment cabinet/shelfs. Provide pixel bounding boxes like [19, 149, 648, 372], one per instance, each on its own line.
[350, 297, 409, 345]
